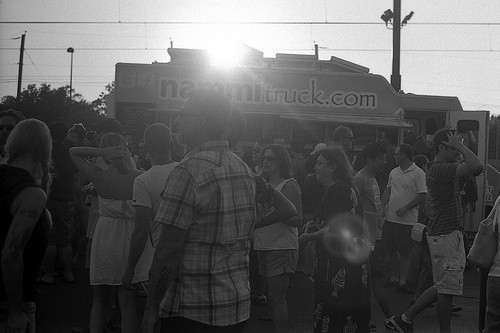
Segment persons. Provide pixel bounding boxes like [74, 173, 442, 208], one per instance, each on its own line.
[0, 88, 500, 333]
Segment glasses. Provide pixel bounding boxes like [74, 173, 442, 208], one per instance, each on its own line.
[178, 108, 204, 122]
[343, 136, 356, 141]
[0, 124, 15, 131]
[260, 155, 275, 163]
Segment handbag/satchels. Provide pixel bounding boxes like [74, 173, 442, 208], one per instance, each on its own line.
[466, 194, 500, 270]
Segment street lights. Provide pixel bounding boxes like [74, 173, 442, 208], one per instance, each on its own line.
[65, 46, 75, 120]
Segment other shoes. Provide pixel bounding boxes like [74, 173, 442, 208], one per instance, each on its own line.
[252, 292, 268, 304]
[391, 312, 413, 333]
[384, 317, 400, 333]
[375, 268, 463, 311]
[131, 282, 148, 296]
[343, 318, 377, 330]
[35, 252, 90, 283]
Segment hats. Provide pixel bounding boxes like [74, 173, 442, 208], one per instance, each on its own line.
[434, 129, 464, 149]
[363, 142, 388, 156]
[310, 143, 326, 155]
[273, 138, 282, 143]
[248, 138, 273, 151]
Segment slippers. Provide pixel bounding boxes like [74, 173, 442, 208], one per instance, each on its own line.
[259, 312, 273, 321]
